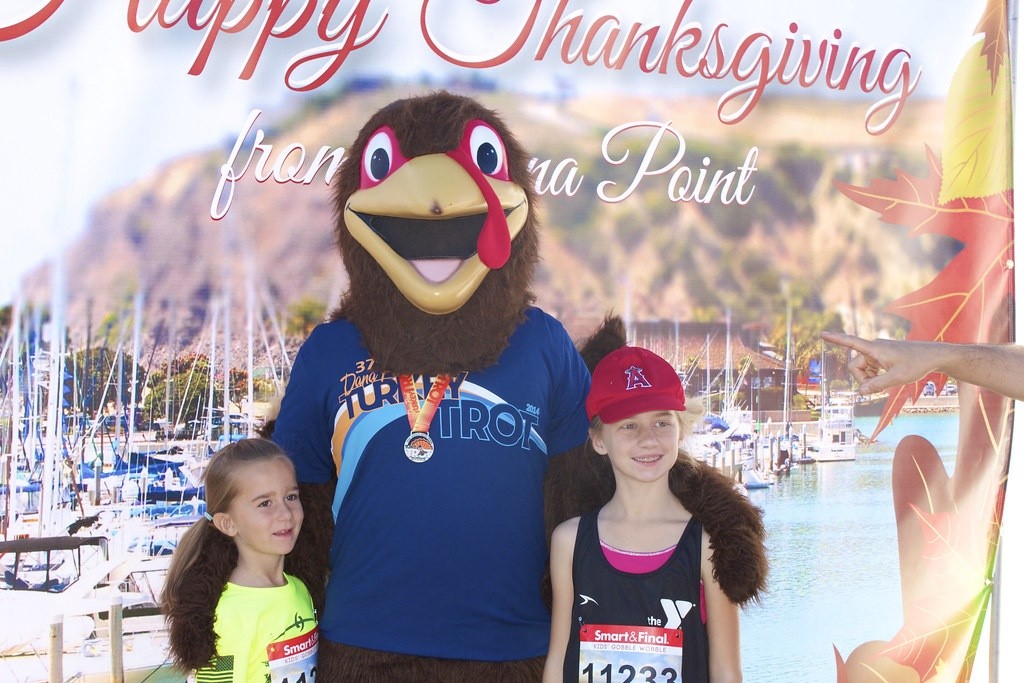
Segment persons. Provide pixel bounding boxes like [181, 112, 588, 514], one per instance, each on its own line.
[542, 346, 743, 683]
[162, 438, 319, 683]
[821, 330, 1024, 402]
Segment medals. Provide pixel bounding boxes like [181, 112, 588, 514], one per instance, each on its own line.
[404, 429, 434, 463]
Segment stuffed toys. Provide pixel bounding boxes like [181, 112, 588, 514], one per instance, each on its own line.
[161, 90, 771, 683]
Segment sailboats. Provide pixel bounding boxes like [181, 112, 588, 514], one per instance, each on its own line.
[1, 201, 863, 619]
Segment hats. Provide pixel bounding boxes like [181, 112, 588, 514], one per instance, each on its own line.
[585, 346, 686, 425]
